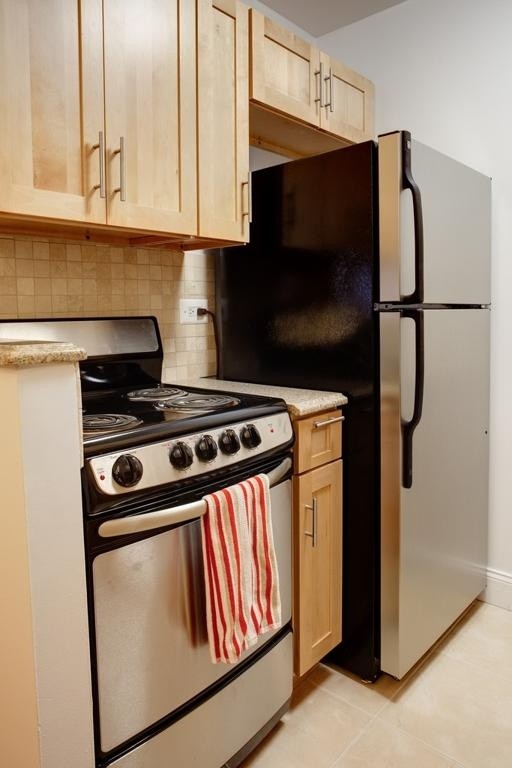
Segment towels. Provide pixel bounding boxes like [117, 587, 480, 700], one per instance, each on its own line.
[200, 472, 281, 665]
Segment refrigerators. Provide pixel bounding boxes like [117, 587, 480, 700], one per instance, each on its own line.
[217, 126, 495, 685]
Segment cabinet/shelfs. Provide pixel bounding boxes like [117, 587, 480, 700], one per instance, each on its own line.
[0, 0, 196, 246]
[145, 0, 252, 252]
[294, 410, 346, 679]
[250, 8, 376, 161]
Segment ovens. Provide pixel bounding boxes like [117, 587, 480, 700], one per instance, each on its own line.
[83, 452, 296, 767]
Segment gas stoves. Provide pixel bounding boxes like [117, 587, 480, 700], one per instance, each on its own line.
[82, 381, 298, 516]
